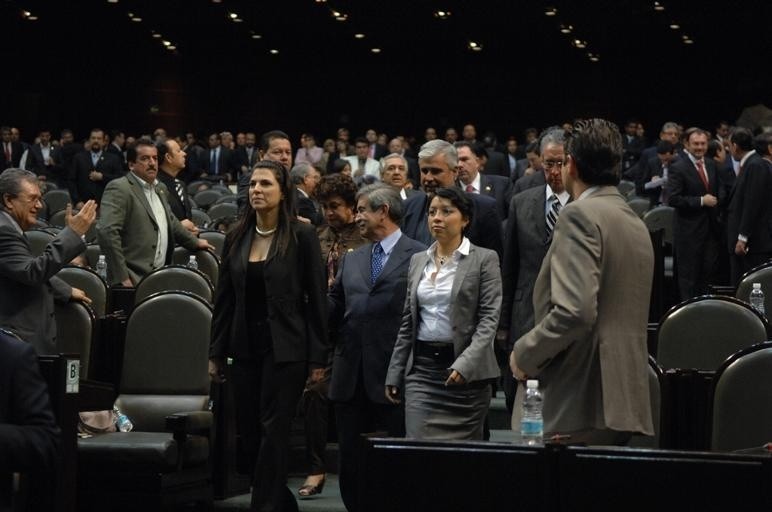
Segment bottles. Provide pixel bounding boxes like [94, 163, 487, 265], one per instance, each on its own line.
[96, 255, 107, 282]
[519, 378, 546, 448]
[187, 255, 199, 269]
[114, 409, 133, 433]
[750, 283, 765, 315]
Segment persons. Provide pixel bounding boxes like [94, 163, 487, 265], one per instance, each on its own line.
[385, 186, 503, 443]
[332, 182, 429, 438]
[205, 159, 330, 512]
[294, 173, 372, 496]
[1, 120, 772, 287]
[505, 120, 656, 449]
[0, 167, 97, 366]
[499, 128, 574, 415]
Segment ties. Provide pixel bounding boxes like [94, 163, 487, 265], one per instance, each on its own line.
[174, 178, 185, 205]
[370, 242, 383, 287]
[464, 185, 475, 194]
[545, 198, 559, 243]
[208, 149, 216, 174]
[696, 159, 710, 193]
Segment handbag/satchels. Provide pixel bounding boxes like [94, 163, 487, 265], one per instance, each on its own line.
[77, 409, 118, 437]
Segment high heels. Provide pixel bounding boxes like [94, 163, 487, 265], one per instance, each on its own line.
[298, 473, 326, 497]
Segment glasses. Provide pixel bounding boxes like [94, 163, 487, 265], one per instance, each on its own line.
[541, 160, 563, 170]
